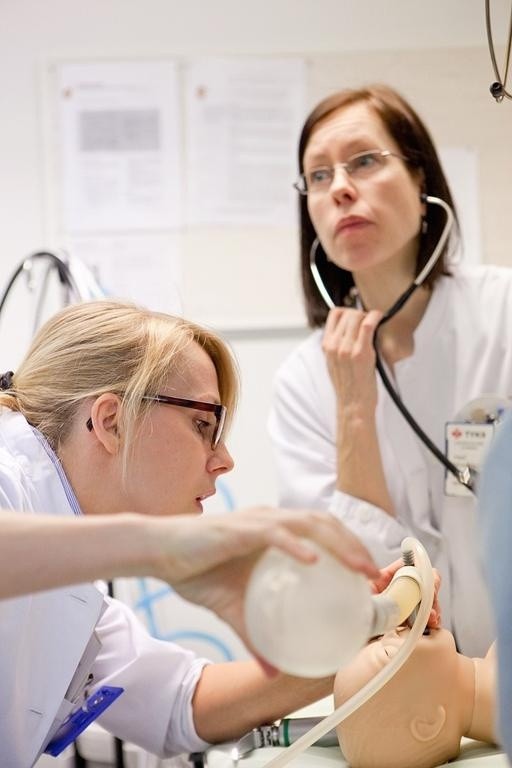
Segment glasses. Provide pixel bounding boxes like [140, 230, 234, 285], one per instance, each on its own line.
[87, 390, 227, 451]
[291, 150, 409, 196]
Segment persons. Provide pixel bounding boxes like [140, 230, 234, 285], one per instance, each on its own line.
[266, 81, 512, 661]
[0, 299, 441, 768]
[334, 628, 497, 767]
